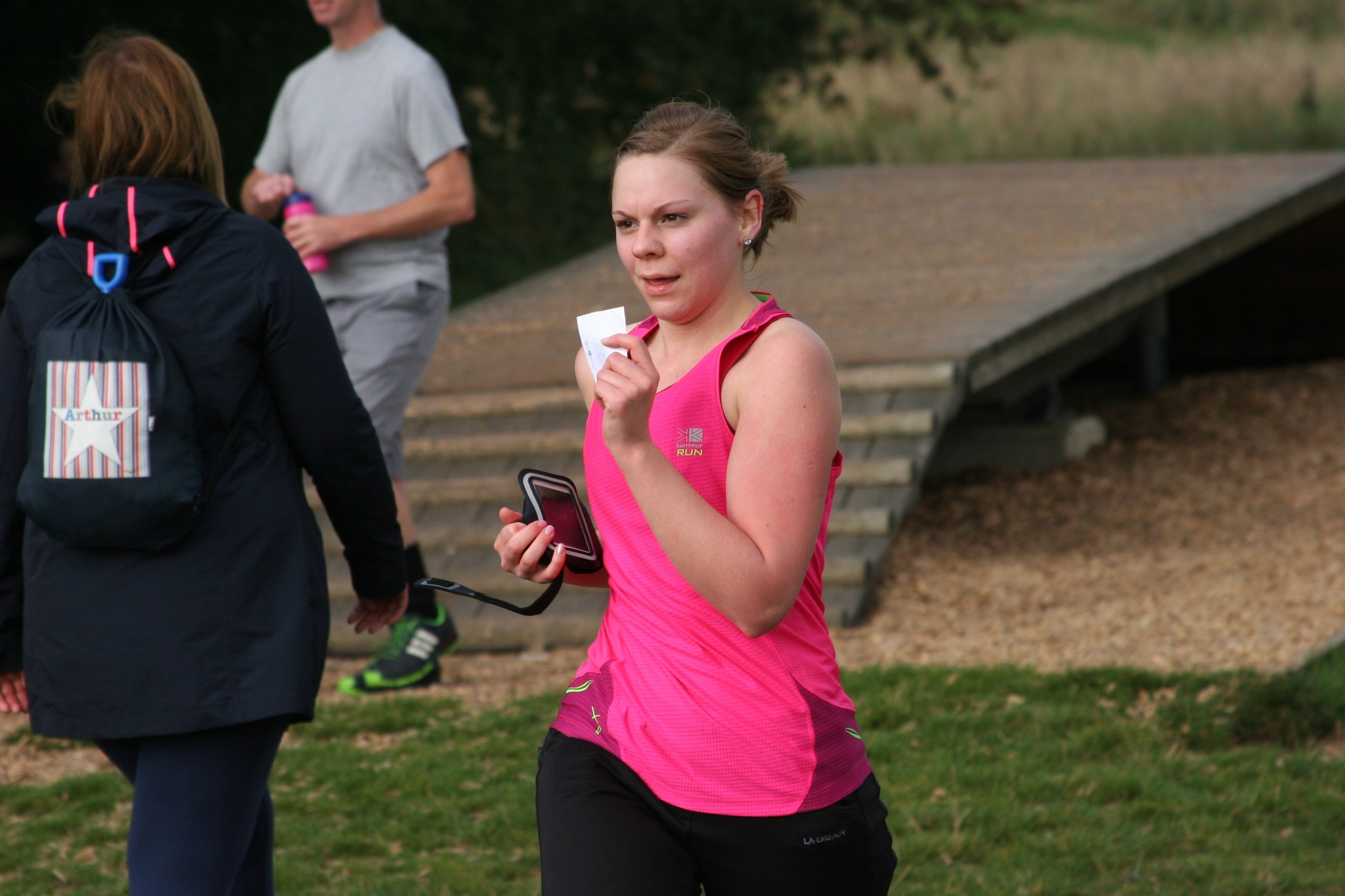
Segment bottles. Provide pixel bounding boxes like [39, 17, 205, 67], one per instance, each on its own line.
[280, 189, 327, 274]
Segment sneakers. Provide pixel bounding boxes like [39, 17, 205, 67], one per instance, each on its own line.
[339, 656, 440, 696]
[363, 602, 459, 686]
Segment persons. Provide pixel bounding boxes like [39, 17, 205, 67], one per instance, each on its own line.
[240, 0, 474, 699]
[494, 101, 899, 896]
[2, 35, 411, 895]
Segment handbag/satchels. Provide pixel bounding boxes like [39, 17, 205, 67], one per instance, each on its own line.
[15, 215, 263, 546]
